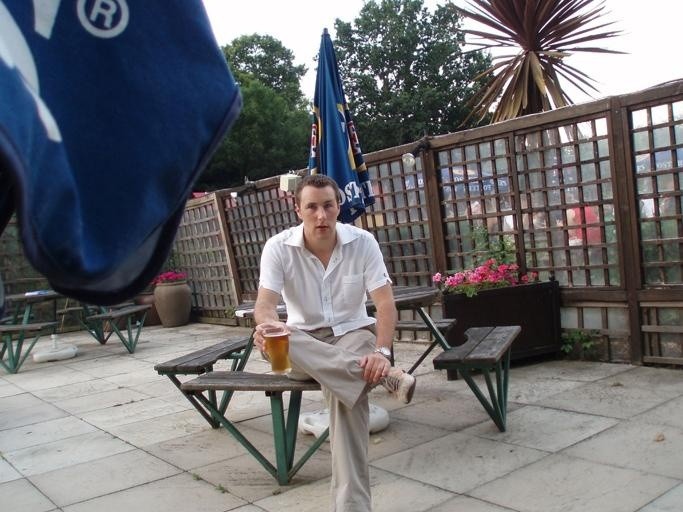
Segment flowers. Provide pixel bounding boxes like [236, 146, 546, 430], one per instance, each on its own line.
[432, 225, 539, 299]
[149, 271, 187, 287]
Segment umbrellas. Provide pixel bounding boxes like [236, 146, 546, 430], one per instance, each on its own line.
[306, 29, 374, 226]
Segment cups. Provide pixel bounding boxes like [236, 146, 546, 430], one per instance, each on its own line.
[261, 331, 292, 375]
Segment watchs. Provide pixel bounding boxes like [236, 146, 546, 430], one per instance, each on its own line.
[373, 346, 392, 360]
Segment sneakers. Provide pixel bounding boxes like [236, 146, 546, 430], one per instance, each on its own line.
[379, 367, 416, 404]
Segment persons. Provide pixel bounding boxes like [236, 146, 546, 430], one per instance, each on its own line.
[251, 173, 416, 512]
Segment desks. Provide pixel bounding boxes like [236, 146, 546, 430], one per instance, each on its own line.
[215, 284, 502, 484]
[0, 290, 131, 373]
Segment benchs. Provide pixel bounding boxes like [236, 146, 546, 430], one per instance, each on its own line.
[153, 338, 255, 429]
[180, 369, 330, 486]
[0, 314, 59, 374]
[432, 323, 522, 432]
[388, 318, 455, 393]
[55, 301, 152, 354]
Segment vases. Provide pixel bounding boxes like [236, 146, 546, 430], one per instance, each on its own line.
[99, 292, 159, 332]
[153, 280, 191, 327]
